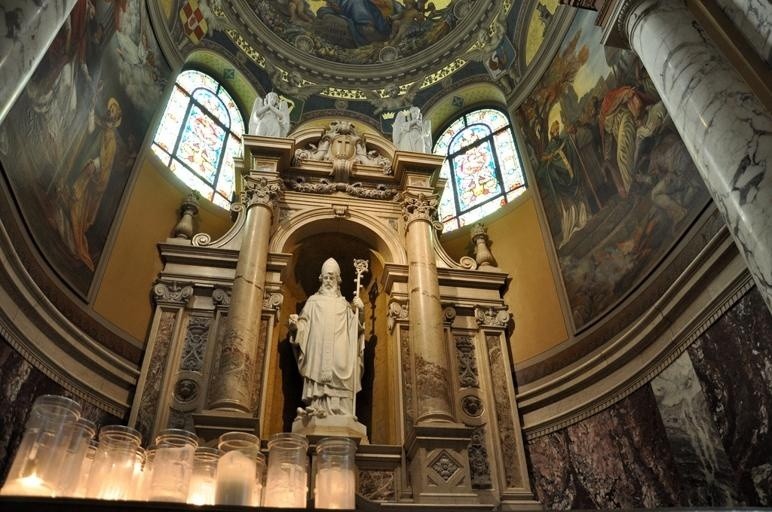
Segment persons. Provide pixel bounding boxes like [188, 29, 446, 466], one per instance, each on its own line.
[542, 80, 693, 251]
[288, 256, 366, 418]
[288, 0, 420, 46]
[402, 106, 426, 153]
[256, 92, 284, 138]
[24, 1, 122, 272]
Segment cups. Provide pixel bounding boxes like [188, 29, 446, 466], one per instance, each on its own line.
[0, 394, 357, 509]
